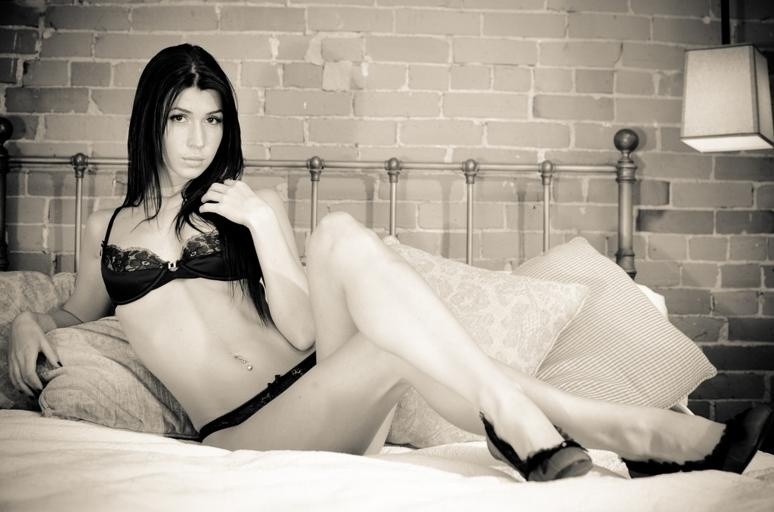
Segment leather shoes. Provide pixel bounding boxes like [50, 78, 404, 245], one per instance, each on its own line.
[481, 413, 592, 480]
[623, 406, 771, 475]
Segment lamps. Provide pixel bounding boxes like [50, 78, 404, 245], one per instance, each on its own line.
[676, 0, 773, 153]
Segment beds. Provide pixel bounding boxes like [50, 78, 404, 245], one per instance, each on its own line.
[0, 117, 773, 510]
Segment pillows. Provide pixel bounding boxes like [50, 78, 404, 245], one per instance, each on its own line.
[510, 237, 716, 414]
[378, 228, 588, 446]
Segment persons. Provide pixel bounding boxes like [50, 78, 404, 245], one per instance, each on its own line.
[6, 44, 771, 481]
